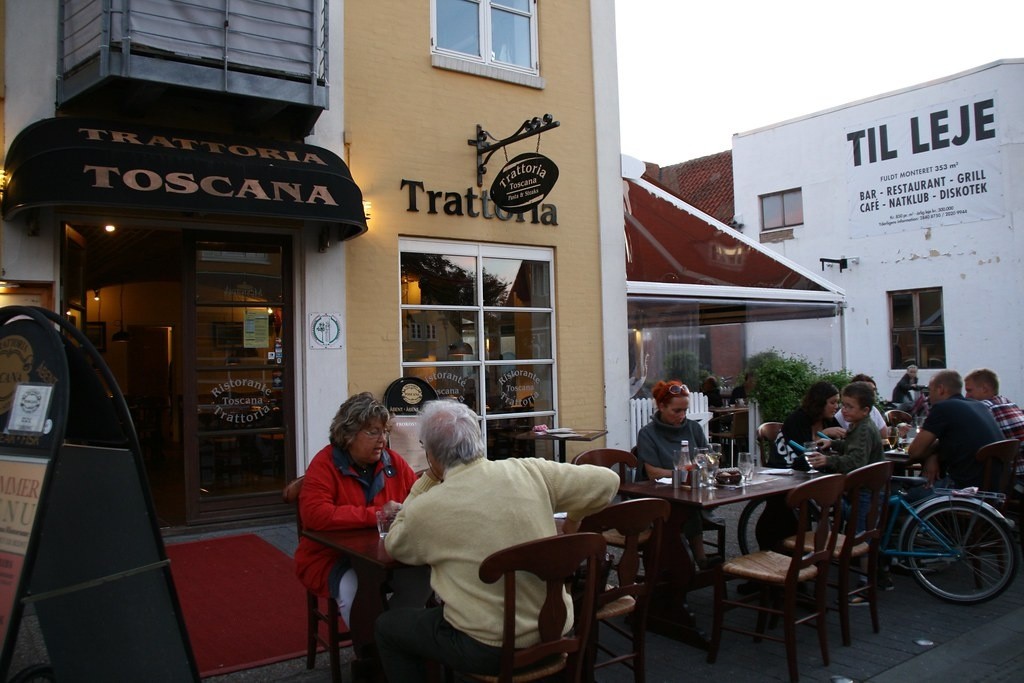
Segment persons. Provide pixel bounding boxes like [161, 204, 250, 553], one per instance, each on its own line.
[728, 371, 757, 424]
[290, 396, 620, 683]
[634, 380, 723, 579]
[764, 364, 1024, 608]
[699, 376, 723, 450]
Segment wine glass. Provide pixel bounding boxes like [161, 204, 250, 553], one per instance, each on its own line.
[887, 426, 898, 452]
[805, 441, 819, 473]
[738, 452, 754, 486]
[673, 442, 721, 489]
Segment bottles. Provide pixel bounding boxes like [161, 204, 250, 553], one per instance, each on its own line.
[678, 440, 693, 490]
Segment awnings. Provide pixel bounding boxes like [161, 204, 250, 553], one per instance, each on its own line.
[0, 113, 369, 253]
[622, 171, 846, 330]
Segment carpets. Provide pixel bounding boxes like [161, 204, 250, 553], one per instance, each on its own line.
[161, 532, 351, 678]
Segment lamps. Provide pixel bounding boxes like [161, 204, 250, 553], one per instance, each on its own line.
[112, 286, 128, 343]
[447, 342, 474, 356]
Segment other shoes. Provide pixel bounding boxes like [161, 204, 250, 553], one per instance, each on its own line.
[699, 555, 722, 569]
[737, 581, 775, 599]
[878, 575, 896, 590]
[847, 591, 867, 606]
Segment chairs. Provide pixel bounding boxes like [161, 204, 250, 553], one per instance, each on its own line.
[575, 498, 672, 683]
[699, 515, 730, 597]
[705, 472, 845, 683]
[709, 410, 749, 467]
[757, 420, 833, 530]
[478, 531, 606, 683]
[780, 460, 896, 645]
[571, 447, 653, 583]
[284, 475, 352, 683]
[923, 437, 1021, 590]
[883, 409, 921, 476]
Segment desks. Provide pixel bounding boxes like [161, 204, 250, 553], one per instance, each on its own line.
[517, 429, 609, 463]
[707, 404, 750, 432]
[305, 526, 407, 615]
[615, 466, 831, 650]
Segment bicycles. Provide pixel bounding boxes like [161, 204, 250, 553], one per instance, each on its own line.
[738, 430, 1020, 605]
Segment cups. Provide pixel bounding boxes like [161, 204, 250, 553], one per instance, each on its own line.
[903, 439, 912, 454]
[376, 510, 397, 537]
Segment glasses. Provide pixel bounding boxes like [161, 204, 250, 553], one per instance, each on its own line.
[838, 402, 865, 410]
[660, 384, 689, 402]
[419, 440, 428, 449]
[361, 429, 389, 439]
